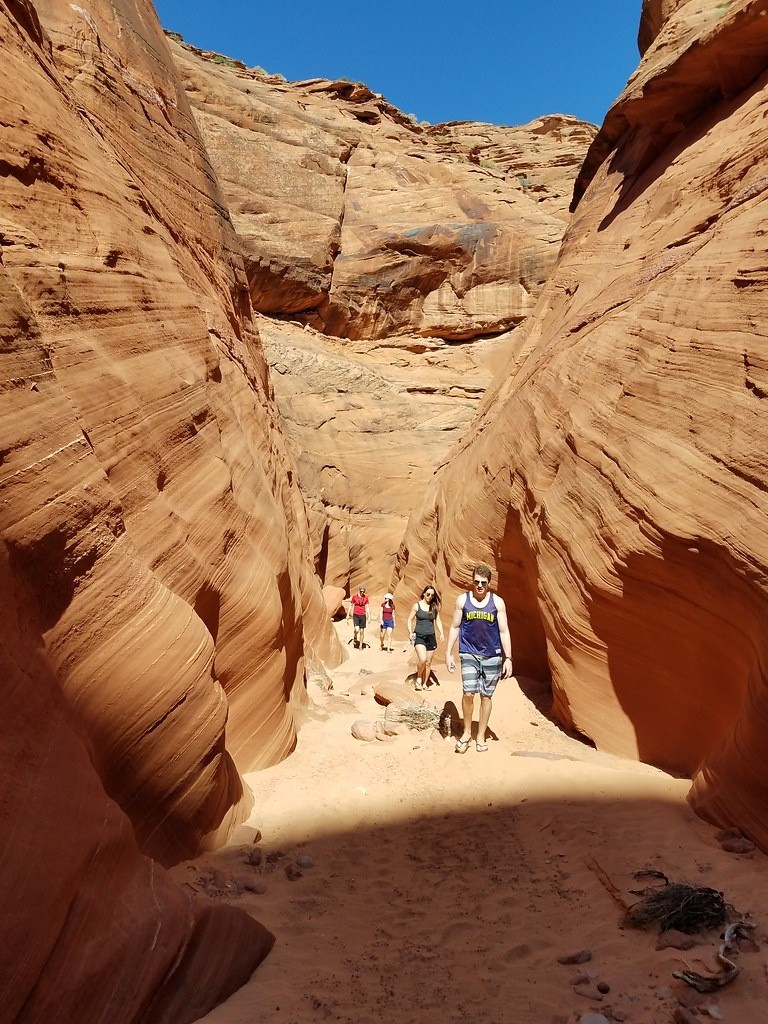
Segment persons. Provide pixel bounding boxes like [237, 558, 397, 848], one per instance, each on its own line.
[346, 586, 371, 649]
[380, 593, 397, 653]
[445, 565, 513, 754]
[406, 585, 445, 691]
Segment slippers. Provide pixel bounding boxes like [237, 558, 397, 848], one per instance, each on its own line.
[455, 736, 472, 754]
[475, 737, 488, 752]
[415, 681, 422, 690]
[423, 687, 432, 691]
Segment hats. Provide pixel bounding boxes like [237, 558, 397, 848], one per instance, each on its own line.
[384, 593, 393, 600]
[359, 586, 366, 591]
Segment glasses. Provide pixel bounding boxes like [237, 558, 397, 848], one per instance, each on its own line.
[425, 593, 435, 597]
[474, 581, 489, 586]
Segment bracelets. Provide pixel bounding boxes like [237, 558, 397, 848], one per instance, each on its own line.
[505, 657, 512, 661]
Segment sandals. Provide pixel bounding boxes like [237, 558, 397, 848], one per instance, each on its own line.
[354, 636, 358, 646]
